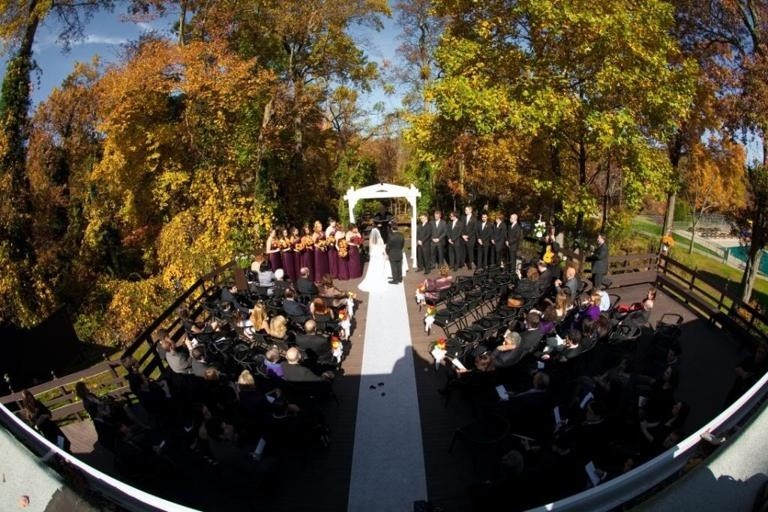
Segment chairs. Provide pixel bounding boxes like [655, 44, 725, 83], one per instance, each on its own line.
[154, 271, 349, 452]
[419, 264, 685, 448]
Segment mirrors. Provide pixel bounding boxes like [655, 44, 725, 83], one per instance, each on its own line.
[0, 1, 767, 512]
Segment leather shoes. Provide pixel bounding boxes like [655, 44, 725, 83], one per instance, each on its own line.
[389, 279, 403, 284]
[415, 263, 483, 275]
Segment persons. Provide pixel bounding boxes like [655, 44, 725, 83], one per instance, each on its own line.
[76, 268, 356, 498]
[21, 390, 73, 451]
[439, 287, 690, 475]
[266, 210, 609, 293]
[725, 342, 768, 408]
